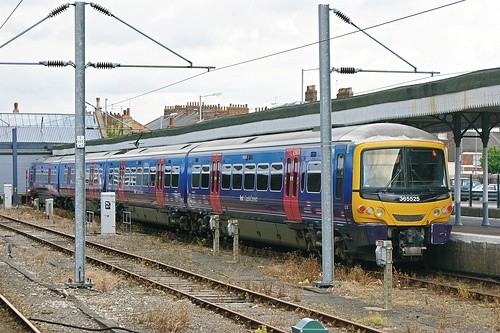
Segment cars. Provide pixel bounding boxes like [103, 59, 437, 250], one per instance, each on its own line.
[471, 184, 499, 200]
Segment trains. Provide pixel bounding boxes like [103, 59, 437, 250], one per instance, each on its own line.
[29, 120, 455, 269]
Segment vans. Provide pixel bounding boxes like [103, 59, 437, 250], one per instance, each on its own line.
[447, 178, 481, 200]
